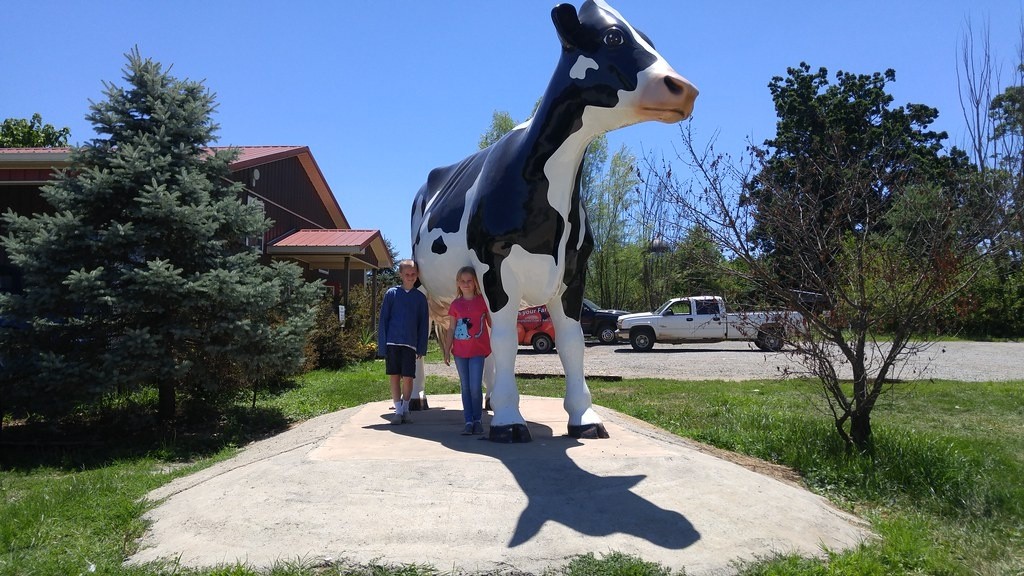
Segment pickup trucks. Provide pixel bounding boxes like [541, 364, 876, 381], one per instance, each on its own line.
[581, 299, 633, 345]
[615, 294, 808, 352]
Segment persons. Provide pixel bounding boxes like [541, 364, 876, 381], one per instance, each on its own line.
[377, 259, 429, 426]
[443, 266, 492, 435]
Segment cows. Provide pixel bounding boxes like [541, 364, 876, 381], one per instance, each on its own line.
[408, 0, 701, 444]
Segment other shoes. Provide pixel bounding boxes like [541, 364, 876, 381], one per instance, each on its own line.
[461, 424, 473, 434]
[402, 412, 414, 423]
[390, 414, 403, 424]
[472, 420, 485, 435]
